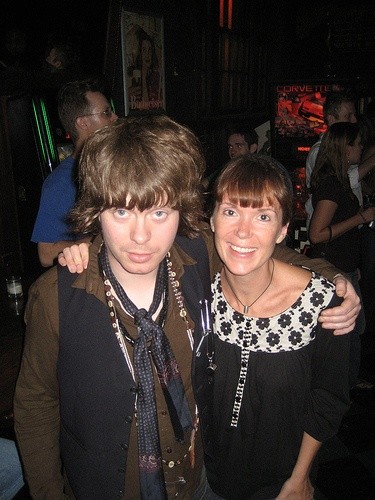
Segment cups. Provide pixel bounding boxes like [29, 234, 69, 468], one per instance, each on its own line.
[5, 276, 24, 299]
[362, 194, 375, 209]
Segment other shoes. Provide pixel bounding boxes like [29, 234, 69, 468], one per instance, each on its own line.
[350, 378, 374, 390]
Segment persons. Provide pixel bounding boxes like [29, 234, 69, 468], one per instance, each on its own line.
[228, 128, 258, 160]
[11, 116, 360, 500]
[305, 92, 375, 237]
[31, 79, 118, 267]
[58, 154, 353, 500]
[310, 122, 375, 390]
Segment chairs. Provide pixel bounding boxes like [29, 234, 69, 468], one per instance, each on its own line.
[0, 438, 25, 500]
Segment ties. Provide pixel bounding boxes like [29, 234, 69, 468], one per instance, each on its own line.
[100, 242, 194, 500]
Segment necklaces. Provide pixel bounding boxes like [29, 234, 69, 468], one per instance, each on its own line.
[113, 259, 166, 344]
[98, 239, 199, 467]
[224, 258, 274, 313]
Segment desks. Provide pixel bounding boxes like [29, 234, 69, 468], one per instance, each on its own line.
[0, 307, 26, 421]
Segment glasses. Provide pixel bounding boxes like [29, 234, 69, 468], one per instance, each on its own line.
[76, 108, 113, 120]
